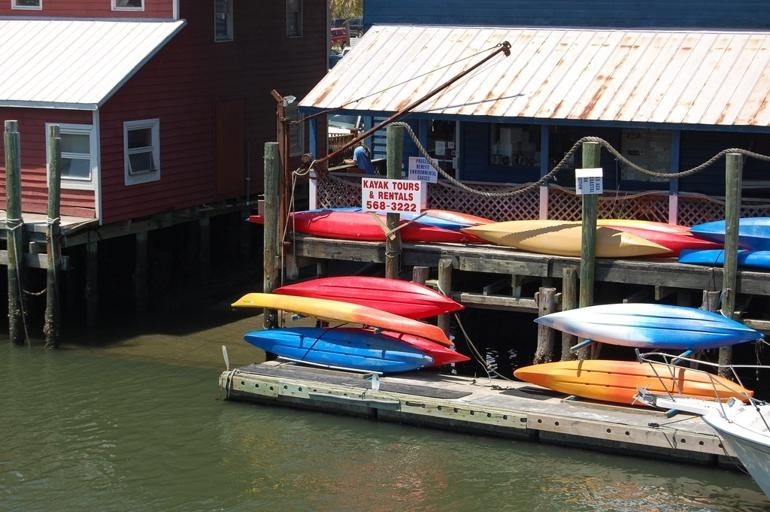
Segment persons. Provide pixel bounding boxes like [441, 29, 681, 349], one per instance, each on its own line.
[343, 137, 375, 177]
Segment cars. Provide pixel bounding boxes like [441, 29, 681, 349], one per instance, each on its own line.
[330, 13, 362, 67]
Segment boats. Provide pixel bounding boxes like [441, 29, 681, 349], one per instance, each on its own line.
[230, 275, 473, 378]
[533, 302, 763, 352]
[634, 340, 769, 499]
[513, 355, 752, 409]
[685, 217, 770, 251]
[675, 247, 768, 272]
[243, 207, 500, 245]
[586, 218, 728, 257]
[457, 219, 674, 259]
